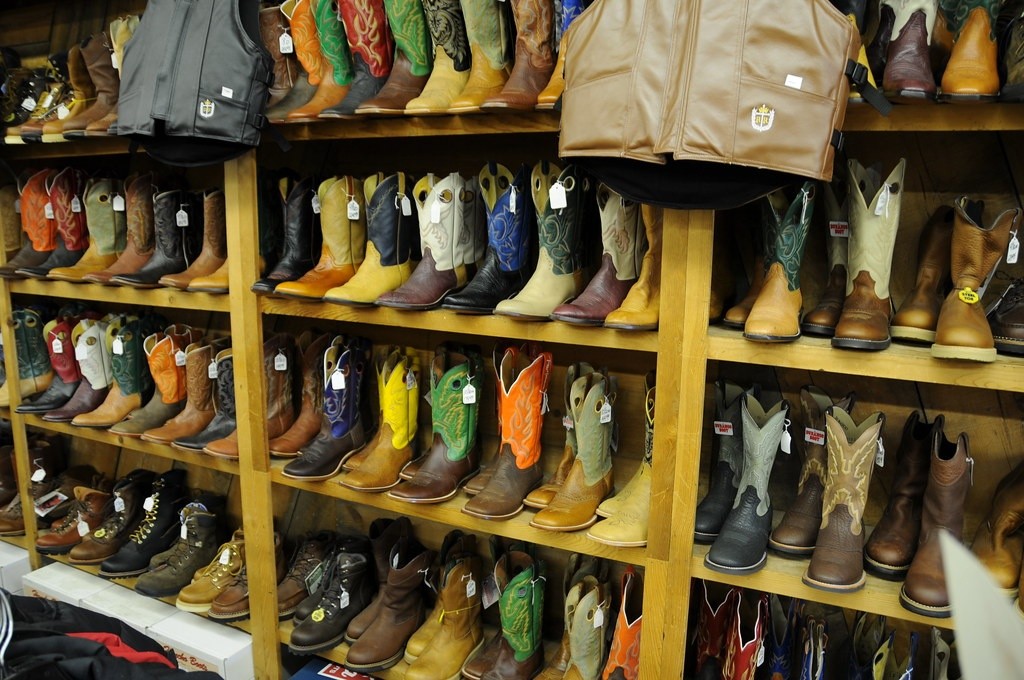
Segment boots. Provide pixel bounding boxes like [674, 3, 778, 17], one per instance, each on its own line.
[0, 0, 1024, 680]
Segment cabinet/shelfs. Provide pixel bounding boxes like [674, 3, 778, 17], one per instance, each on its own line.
[0, 45, 1024, 679]
